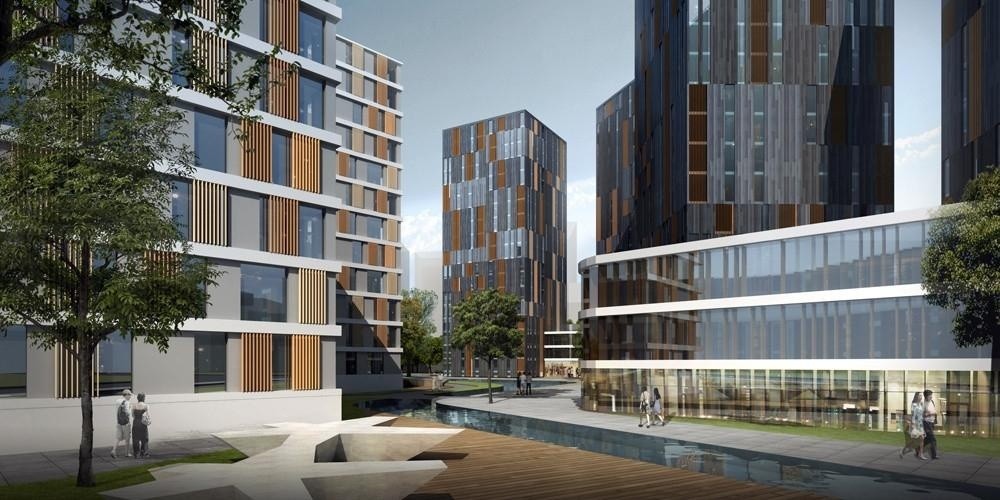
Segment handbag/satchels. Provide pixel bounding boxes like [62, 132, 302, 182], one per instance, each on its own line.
[639, 400, 648, 413]
[934, 413, 938, 424]
[141, 405, 152, 426]
[117, 400, 130, 425]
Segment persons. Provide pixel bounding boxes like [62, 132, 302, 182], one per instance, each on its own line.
[131, 391, 152, 459]
[651, 387, 666, 427]
[515, 372, 521, 395]
[110, 389, 133, 458]
[589, 380, 599, 412]
[638, 386, 651, 428]
[545, 364, 579, 378]
[914, 389, 939, 459]
[519, 372, 526, 395]
[526, 372, 532, 395]
[899, 391, 927, 460]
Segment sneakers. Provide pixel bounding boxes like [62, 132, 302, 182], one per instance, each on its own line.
[110, 452, 117, 459]
[125, 453, 132, 457]
[133, 453, 144, 456]
[652, 421, 665, 426]
[899, 449, 940, 460]
[638, 424, 650, 428]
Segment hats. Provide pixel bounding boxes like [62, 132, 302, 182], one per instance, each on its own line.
[122, 389, 133, 395]
